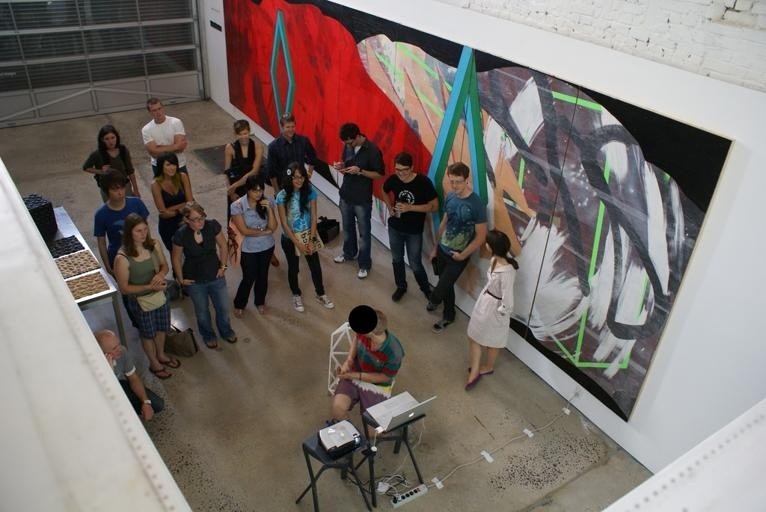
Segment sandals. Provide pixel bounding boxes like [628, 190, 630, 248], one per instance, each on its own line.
[219, 335, 237, 343]
[205, 339, 218, 349]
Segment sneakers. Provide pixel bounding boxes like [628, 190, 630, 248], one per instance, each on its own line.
[292, 295, 304, 313]
[392, 287, 456, 332]
[232, 303, 245, 319]
[357, 268, 368, 278]
[333, 254, 358, 263]
[315, 294, 334, 308]
[257, 305, 266, 315]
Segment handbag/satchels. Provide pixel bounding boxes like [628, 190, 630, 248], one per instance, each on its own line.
[163, 323, 199, 356]
[226, 217, 245, 268]
[316, 216, 339, 244]
[136, 290, 168, 312]
[294, 227, 324, 256]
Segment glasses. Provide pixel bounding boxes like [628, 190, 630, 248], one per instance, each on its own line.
[395, 167, 411, 172]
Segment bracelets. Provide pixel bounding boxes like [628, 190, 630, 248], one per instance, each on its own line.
[359, 372, 362, 381]
[142, 399, 152, 404]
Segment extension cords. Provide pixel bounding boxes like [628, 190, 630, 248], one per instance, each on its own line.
[390, 484, 427, 507]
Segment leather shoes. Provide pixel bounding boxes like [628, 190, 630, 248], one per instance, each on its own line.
[465, 371, 480, 391]
[468, 366, 494, 375]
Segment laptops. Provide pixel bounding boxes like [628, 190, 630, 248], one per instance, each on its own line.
[366, 391, 437, 432]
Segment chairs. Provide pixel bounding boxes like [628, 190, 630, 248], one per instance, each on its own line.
[355, 400, 426, 508]
[293, 415, 374, 511]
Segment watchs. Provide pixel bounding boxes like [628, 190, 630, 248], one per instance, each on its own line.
[357, 169, 362, 176]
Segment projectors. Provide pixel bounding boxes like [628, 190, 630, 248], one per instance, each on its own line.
[319, 420, 363, 458]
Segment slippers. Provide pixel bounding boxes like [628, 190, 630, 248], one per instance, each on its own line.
[148, 365, 172, 379]
[158, 355, 181, 368]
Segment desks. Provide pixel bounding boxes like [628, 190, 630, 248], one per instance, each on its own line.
[40, 205, 129, 355]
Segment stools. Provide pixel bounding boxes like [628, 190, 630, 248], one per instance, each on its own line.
[162, 306, 200, 355]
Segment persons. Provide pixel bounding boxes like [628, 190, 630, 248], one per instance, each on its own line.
[225, 113, 318, 266]
[426, 161, 488, 333]
[233, 175, 278, 319]
[464, 229, 518, 390]
[332, 121, 385, 278]
[140, 96, 188, 175]
[381, 152, 439, 303]
[172, 200, 238, 379]
[97, 329, 165, 421]
[329, 311, 405, 438]
[277, 162, 334, 313]
[83, 125, 140, 204]
[94, 172, 150, 278]
[150, 151, 197, 283]
[114, 212, 180, 379]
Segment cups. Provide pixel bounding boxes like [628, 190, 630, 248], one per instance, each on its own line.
[395, 203, 402, 219]
